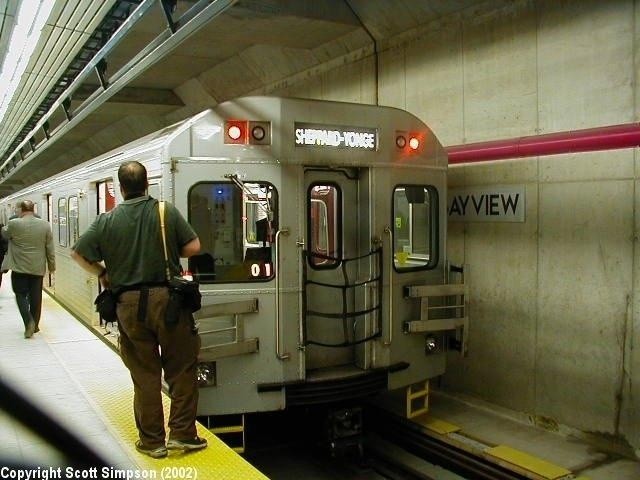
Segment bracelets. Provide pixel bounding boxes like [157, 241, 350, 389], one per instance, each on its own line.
[97, 269, 107, 277]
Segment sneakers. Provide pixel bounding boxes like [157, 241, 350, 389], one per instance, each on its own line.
[135, 438, 168, 459]
[166, 437, 208, 453]
[23, 322, 40, 339]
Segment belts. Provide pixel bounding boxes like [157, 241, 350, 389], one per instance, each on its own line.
[115, 278, 170, 323]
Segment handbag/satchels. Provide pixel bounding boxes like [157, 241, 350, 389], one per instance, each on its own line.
[92, 289, 120, 329]
[166, 278, 204, 314]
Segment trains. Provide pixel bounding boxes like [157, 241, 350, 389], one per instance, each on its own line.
[0, 91, 472, 463]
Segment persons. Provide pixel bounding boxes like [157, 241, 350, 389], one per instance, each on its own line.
[0, 199, 57, 339]
[69, 160, 208, 459]
